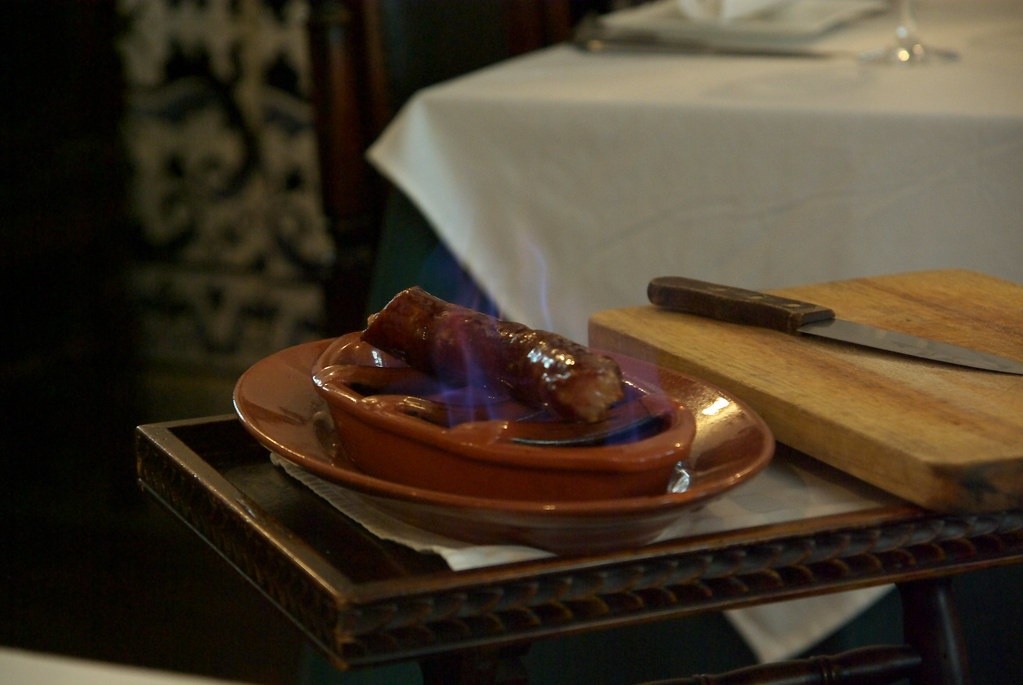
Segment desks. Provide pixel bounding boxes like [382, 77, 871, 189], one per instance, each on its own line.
[365, 0, 1023, 685]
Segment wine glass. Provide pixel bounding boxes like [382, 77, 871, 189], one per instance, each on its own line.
[862, 1, 964, 69]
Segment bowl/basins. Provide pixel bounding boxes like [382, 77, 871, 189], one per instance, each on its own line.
[310, 329, 696, 504]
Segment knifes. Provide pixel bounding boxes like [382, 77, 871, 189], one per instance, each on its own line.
[569, 21, 960, 59]
[647, 274, 1023, 374]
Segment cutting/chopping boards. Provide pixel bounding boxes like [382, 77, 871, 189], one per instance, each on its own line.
[588, 268, 1023, 515]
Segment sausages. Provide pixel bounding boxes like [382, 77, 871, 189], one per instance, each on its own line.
[357, 288, 624, 425]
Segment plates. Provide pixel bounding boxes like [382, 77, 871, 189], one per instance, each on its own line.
[233, 336, 776, 529]
